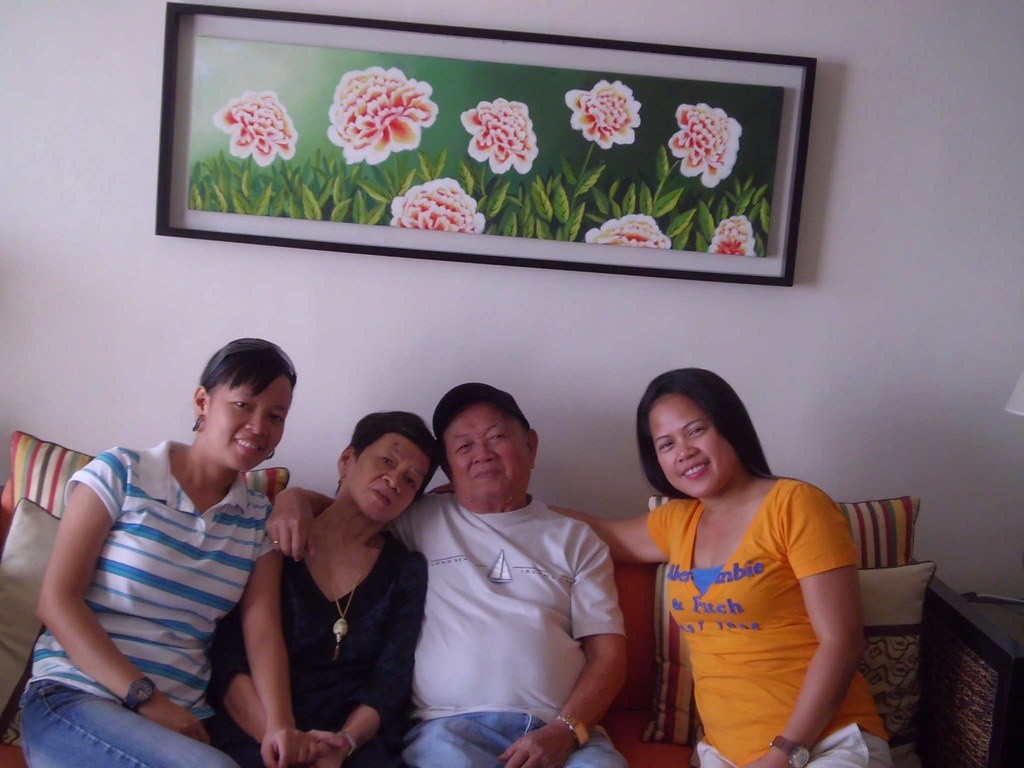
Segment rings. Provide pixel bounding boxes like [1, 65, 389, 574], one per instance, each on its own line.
[272, 541, 280, 544]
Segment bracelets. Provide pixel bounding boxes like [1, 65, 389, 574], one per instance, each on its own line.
[338, 731, 357, 756]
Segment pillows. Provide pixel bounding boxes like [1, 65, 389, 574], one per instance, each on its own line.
[0, 431, 289, 742]
[641, 498, 940, 760]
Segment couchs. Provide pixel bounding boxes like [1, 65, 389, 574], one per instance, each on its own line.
[0, 523, 1024, 768]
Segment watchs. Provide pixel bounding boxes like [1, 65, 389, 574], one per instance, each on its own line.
[770, 735, 810, 768]
[126, 677, 156, 711]
[556, 712, 590, 748]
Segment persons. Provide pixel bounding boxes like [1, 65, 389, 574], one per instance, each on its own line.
[208, 411, 441, 768]
[265, 382, 630, 768]
[20, 338, 320, 768]
[425, 367, 892, 768]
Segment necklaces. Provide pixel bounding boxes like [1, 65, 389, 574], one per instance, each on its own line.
[325, 540, 374, 662]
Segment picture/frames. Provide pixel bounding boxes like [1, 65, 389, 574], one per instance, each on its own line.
[157, 1, 818, 287]
